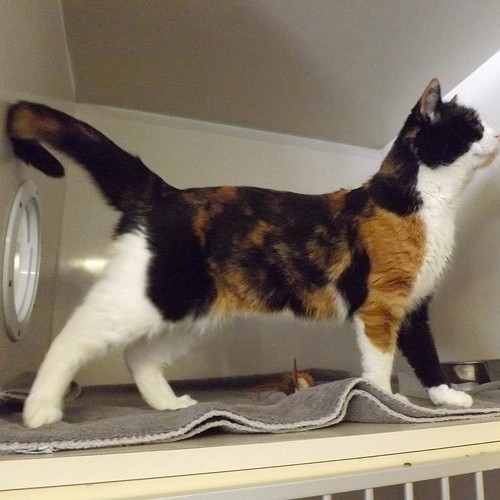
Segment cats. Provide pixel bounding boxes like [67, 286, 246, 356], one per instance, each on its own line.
[5, 75, 499, 429]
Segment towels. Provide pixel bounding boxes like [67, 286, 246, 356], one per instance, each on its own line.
[0, 359, 500, 457]
